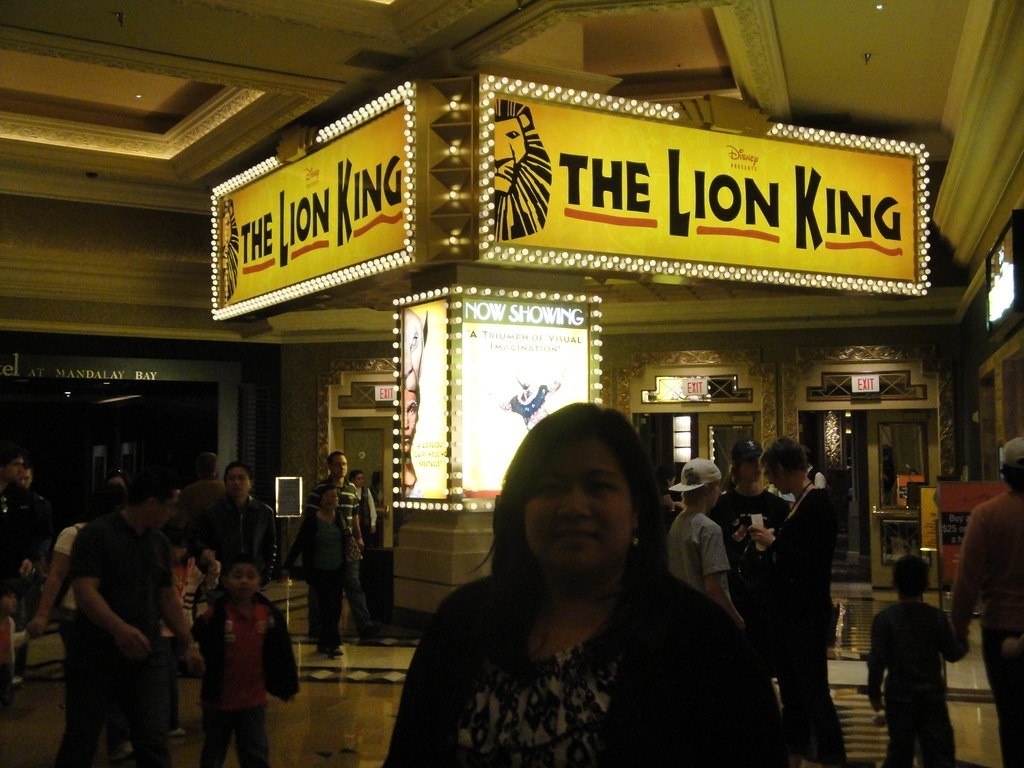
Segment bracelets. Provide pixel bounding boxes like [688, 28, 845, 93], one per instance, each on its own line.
[186, 642, 200, 651]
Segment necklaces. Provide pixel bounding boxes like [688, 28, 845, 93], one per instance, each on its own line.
[787, 481, 810, 517]
[283, 487, 361, 658]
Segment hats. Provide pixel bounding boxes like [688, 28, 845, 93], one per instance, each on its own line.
[730, 438, 764, 460]
[1002, 436, 1024, 468]
[668, 458, 722, 492]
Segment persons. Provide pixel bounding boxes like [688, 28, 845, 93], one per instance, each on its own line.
[382, 402, 782, 767]
[882, 443, 896, 506]
[370, 470, 382, 508]
[866, 555, 969, 768]
[0, 449, 303, 768]
[951, 437, 1024, 765]
[309, 451, 381, 637]
[349, 470, 376, 533]
[644, 440, 847, 768]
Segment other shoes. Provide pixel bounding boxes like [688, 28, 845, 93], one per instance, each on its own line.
[107, 742, 135, 764]
[164, 727, 187, 738]
[316, 639, 343, 658]
